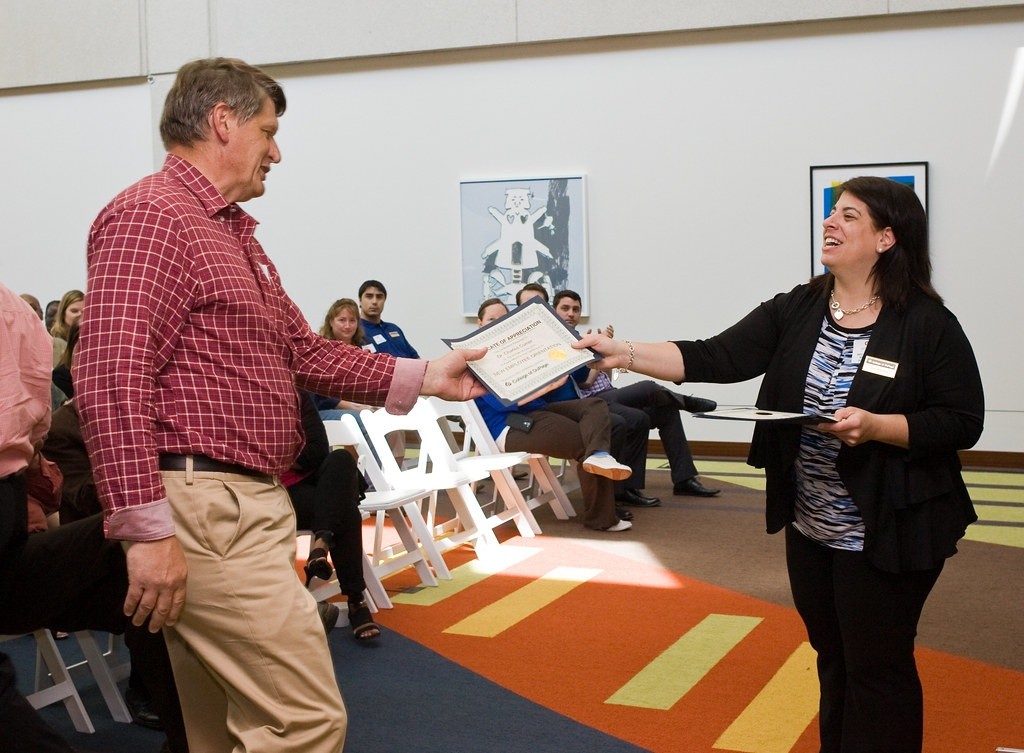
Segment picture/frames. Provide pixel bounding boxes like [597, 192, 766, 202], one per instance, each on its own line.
[459, 176, 591, 320]
[810, 160, 930, 277]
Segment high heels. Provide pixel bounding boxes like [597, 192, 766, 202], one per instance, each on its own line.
[348, 599, 381, 640]
[304, 548, 332, 588]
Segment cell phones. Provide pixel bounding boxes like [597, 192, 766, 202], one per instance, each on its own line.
[506, 412, 535, 433]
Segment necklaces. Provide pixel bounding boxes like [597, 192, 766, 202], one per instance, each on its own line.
[831, 291, 880, 321]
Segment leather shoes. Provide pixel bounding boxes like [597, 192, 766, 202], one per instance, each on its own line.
[674, 479, 721, 496]
[614, 488, 661, 507]
[124, 685, 164, 730]
[683, 395, 717, 413]
[614, 504, 633, 519]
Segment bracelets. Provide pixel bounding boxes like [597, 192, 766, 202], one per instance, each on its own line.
[620, 341, 634, 373]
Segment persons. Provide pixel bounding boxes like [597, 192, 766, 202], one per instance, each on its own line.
[554, 290, 720, 495]
[282, 393, 381, 641]
[0, 281, 188, 752]
[317, 298, 405, 466]
[570, 176, 984, 752]
[359, 280, 528, 491]
[473, 298, 632, 531]
[68, 57, 489, 752]
[516, 283, 660, 517]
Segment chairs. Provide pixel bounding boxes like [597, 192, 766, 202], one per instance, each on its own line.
[0, 628, 133, 733]
[295, 395, 578, 614]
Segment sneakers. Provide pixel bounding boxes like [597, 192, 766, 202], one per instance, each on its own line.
[582, 455, 632, 480]
[606, 520, 632, 532]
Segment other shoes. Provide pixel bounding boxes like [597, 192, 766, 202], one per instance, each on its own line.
[50, 631, 69, 640]
[476, 483, 484, 491]
[512, 469, 528, 478]
[317, 602, 340, 635]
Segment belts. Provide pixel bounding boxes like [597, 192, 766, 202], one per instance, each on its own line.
[158, 452, 270, 478]
